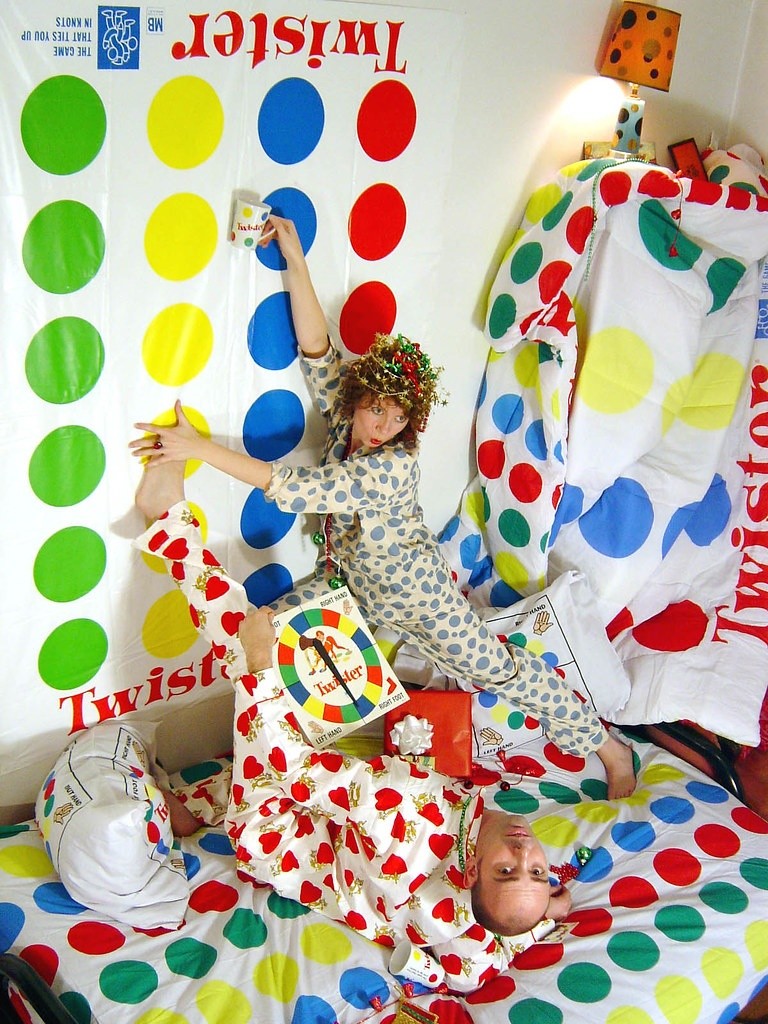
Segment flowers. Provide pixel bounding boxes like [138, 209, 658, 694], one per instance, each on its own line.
[389, 715, 434, 755]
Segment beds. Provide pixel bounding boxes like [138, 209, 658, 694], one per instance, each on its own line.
[1, 717, 768, 1024]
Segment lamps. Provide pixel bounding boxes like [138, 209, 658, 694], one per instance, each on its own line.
[600, 1, 682, 158]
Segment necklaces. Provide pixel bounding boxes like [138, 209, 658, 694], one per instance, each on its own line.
[458, 797, 472, 871]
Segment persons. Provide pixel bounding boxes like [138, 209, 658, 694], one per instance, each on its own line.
[134, 454, 571, 991]
[128, 215, 635, 799]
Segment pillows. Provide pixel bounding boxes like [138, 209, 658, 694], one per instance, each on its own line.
[34, 719, 189, 931]
[393, 570, 631, 759]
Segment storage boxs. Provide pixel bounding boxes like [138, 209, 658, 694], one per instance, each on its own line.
[381, 689, 472, 779]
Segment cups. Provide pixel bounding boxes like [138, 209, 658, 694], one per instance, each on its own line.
[230, 197, 277, 251]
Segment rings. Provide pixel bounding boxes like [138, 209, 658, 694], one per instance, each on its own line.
[154, 438, 162, 449]
